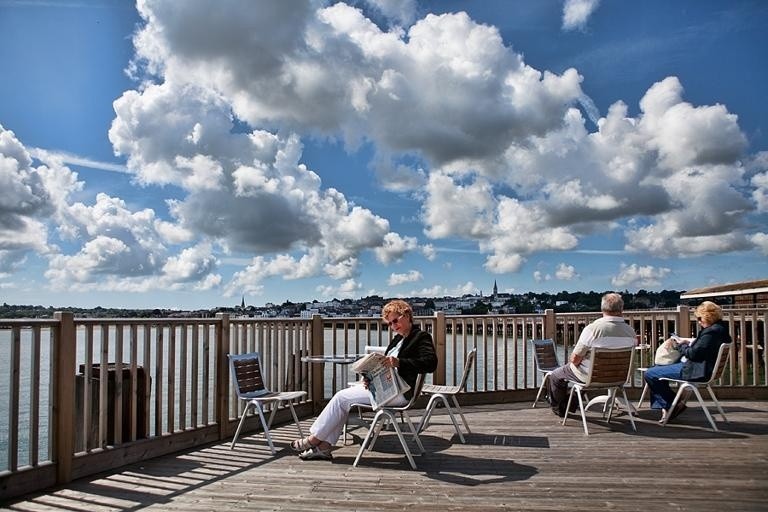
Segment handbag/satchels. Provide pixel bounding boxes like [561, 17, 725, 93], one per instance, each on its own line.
[654, 336, 690, 366]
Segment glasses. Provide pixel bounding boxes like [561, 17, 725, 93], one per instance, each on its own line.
[389, 314, 404, 326]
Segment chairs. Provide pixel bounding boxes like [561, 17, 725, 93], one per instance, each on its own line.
[411, 345, 477, 442]
[351, 352, 428, 470]
[226, 351, 308, 453]
[636, 335, 700, 409]
[532, 337, 573, 407]
[562, 346, 636, 433]
[657, 343, 732, 432]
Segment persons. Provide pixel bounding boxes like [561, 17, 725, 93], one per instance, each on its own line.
[643, 300, 731, 424]
[547, 292, 636, 417]
[288, 299, 437, 462]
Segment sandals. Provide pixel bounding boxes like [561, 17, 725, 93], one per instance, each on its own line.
[290, 437, 333, 460]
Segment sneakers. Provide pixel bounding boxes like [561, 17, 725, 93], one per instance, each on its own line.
[670, 402, 687, 419]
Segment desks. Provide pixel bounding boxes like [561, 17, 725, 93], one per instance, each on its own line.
[300, 353, 388, 423]
[584, 345, 650, 416]
